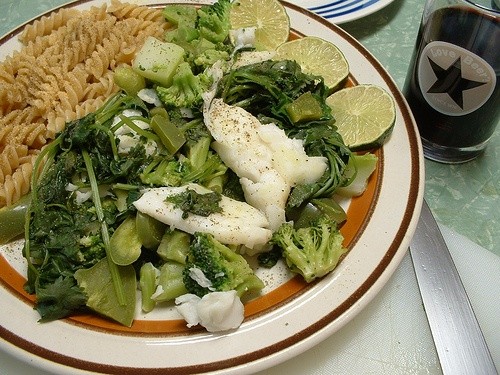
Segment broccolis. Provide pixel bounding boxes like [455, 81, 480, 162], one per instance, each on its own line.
[196, 1, 229, 44]
[156, 63, 213, 105]
[74, 235, 105, 262]
[186, 238, 263, 298]
[273, 221, 348, 280]
[184, 121, 210, 170]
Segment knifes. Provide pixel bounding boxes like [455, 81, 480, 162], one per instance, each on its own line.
[410, 198, 500, 372]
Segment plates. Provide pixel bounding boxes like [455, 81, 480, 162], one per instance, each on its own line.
[0, 1, 426, 375]
[288, 0, 394, 25]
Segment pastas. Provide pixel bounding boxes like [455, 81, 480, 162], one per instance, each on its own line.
[0, 0, 170, 208]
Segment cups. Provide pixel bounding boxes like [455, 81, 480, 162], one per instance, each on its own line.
[401, 0, 500, 165]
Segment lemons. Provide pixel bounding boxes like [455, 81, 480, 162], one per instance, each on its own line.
[275, 37, 349, 90]
[230, 1, 290, 50]
[326, 84, 395, 146]
